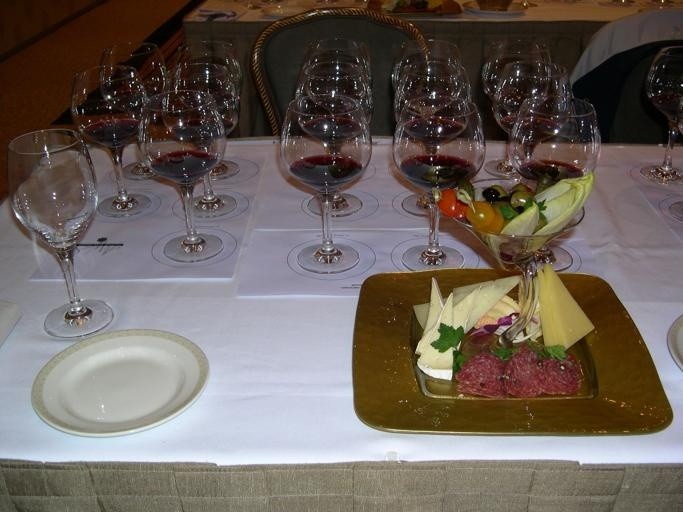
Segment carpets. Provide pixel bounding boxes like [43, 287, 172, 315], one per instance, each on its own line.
[52, 0, 205, 142]
[0, 138, 679, 512]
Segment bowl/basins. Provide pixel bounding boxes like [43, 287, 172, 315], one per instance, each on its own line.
[475, 1, 513, 11]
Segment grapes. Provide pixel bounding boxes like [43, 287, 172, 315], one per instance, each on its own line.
[468, 183, 547, 235]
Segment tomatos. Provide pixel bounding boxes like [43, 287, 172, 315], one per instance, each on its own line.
[437, 188, 467, 219]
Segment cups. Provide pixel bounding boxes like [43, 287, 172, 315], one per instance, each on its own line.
[493, 59, 572, 162]
[393, 40, 462, 86]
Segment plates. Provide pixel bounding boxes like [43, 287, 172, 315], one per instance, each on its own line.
[192, 5, 247, 21]
[463, 1, 528, 16]
[27, 328, 211, 439]
[353, 270, 673, 436]
[262, 2, 312, 21]
[666, 312, 683, 374]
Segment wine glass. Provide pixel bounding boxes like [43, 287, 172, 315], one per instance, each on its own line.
[138, 88, 238, 267]
[392, 60, 472, 224]
[178, 38, 261, 187]
[100, 41, 169, 184]
[4, 129, 116, 340]
[163, 62, 249, 223]
[301, 35, 377, 182]
[480, 37, 553, 179]
[71, 64, 162, 220]
[390, 97, 486, 269]
[506, 94, 603, 271]
[294, 62, 379, 223]
[642, 46, 683, 220]
[281, 94, 378, 280]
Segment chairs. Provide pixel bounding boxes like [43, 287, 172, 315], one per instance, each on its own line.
[251, 7, 430, 137]
[610, 51, 683, 143]
